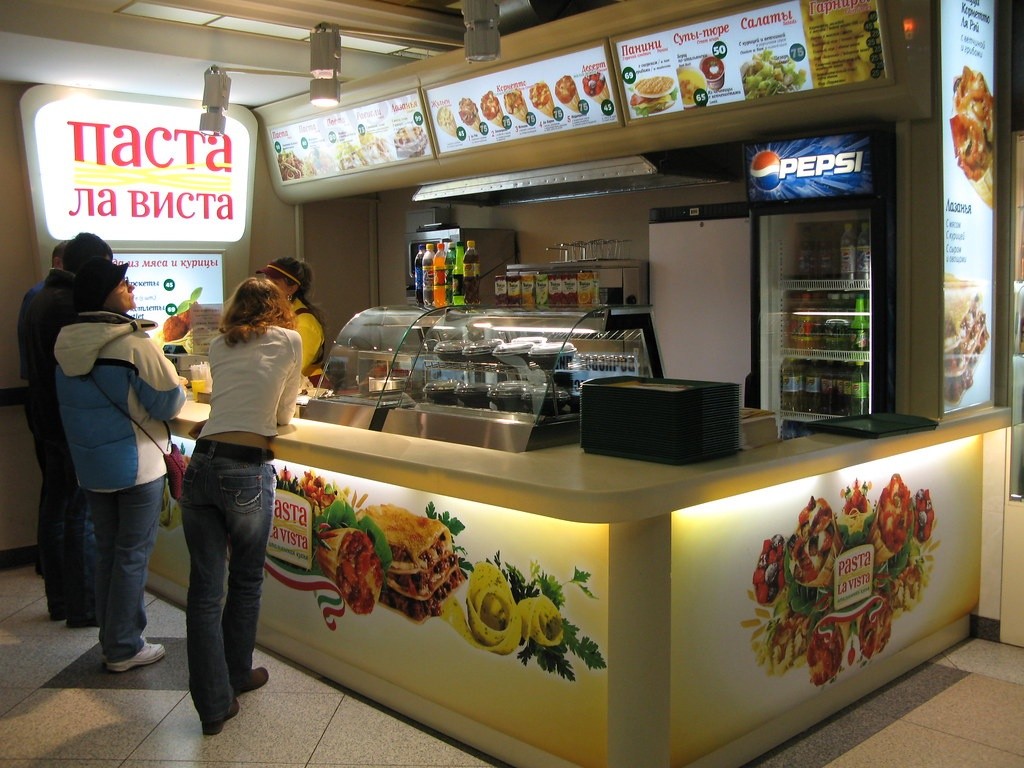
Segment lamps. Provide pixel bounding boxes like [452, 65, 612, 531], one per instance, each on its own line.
[461, 0, 500, 64]
[309, 22, 342, 107]
[199, 65, 232, 137]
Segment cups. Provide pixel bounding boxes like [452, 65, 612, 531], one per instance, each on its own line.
[190, 380, 206, 402]
[700, 55, 725, 91]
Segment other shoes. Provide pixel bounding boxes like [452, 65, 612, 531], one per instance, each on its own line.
[67, 618, 100, 626]
[50, 613, 66, 620]
[234, 667, 269, 696]
[203, 700, 240, 735]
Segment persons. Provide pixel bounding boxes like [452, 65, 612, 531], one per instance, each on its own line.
[255, 257, 329, 388]
[51, 256, 187, 672]
[181, 278, 303, 734]
[17, 232, 113, 627]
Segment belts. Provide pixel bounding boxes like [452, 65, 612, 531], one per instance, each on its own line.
[194, 439, 274, 464]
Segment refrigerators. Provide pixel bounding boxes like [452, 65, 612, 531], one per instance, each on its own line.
[740, 128, 894, 447]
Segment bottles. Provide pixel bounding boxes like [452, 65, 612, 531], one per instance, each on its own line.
[414, 241, 480, 307]
[779, 222, 871, 444]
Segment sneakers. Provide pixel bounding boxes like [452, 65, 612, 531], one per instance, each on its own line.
[101, 635, 147, 664]
[106, 643, 166, 673]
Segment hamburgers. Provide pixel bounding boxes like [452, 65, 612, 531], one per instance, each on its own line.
[628, 76, 678, 118]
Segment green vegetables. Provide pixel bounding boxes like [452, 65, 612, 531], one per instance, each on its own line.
[177, 286, 204, 314]
[743, 49, 807, 99]
[425, 502, 607, 682]
[311, 495, 394, 579]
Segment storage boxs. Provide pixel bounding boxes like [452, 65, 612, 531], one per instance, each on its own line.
[197, 392, 211, 404]
[294, 405, 306, 419]
[369, 376, 412, 392]
[599, 287, 623, 306]
[404, 206, 450, 234]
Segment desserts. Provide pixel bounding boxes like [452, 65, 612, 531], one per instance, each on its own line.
[435, 71, 608, 138]
[749, 474, 939, 685]
[950, 67, 995, 206]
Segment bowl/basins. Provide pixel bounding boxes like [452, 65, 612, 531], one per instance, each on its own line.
[423, 341, 582, 413]
[676, 65, 708, 110]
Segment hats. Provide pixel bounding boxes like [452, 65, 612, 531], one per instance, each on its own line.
[256, 262, 303, 287]
[73, 256, 129, 312]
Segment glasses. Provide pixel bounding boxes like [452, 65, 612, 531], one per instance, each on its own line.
[114, 277, 129, 289]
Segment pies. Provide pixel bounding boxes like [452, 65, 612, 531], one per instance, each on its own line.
[318, 529, 383, 616]
[354, 504, 466, 624]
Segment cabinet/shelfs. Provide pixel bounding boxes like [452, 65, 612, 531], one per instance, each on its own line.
[303, 302, 461, 433]
[380, 304, 667, 455]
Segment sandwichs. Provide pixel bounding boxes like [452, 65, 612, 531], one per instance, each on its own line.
[944, 272, 990, 405]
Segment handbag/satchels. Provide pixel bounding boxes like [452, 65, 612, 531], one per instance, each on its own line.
[163, 443, 185, 499]
